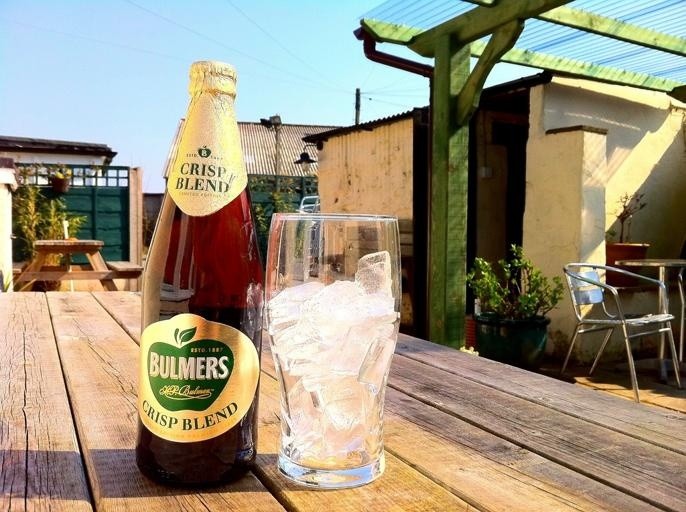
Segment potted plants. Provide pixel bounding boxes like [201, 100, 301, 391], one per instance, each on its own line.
[600, 188, 651, 284]
[465, 241, 565, 369]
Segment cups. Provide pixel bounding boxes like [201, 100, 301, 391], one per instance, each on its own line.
[263, 210, 402, 491]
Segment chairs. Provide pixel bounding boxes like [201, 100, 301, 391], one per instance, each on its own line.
[556, 259, 685, 405]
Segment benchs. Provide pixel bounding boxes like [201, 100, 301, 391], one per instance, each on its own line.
[105, 257, 146, 282]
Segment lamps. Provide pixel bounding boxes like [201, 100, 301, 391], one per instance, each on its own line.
[294, 138, 325, 166]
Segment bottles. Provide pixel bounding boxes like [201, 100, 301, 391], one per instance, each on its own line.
[134, 58, 261, 496]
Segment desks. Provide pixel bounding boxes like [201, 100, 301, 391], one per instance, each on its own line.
[0, 291, 684, 510]
[15, 237, 117, 290]
[611, 259, 686, 379]
